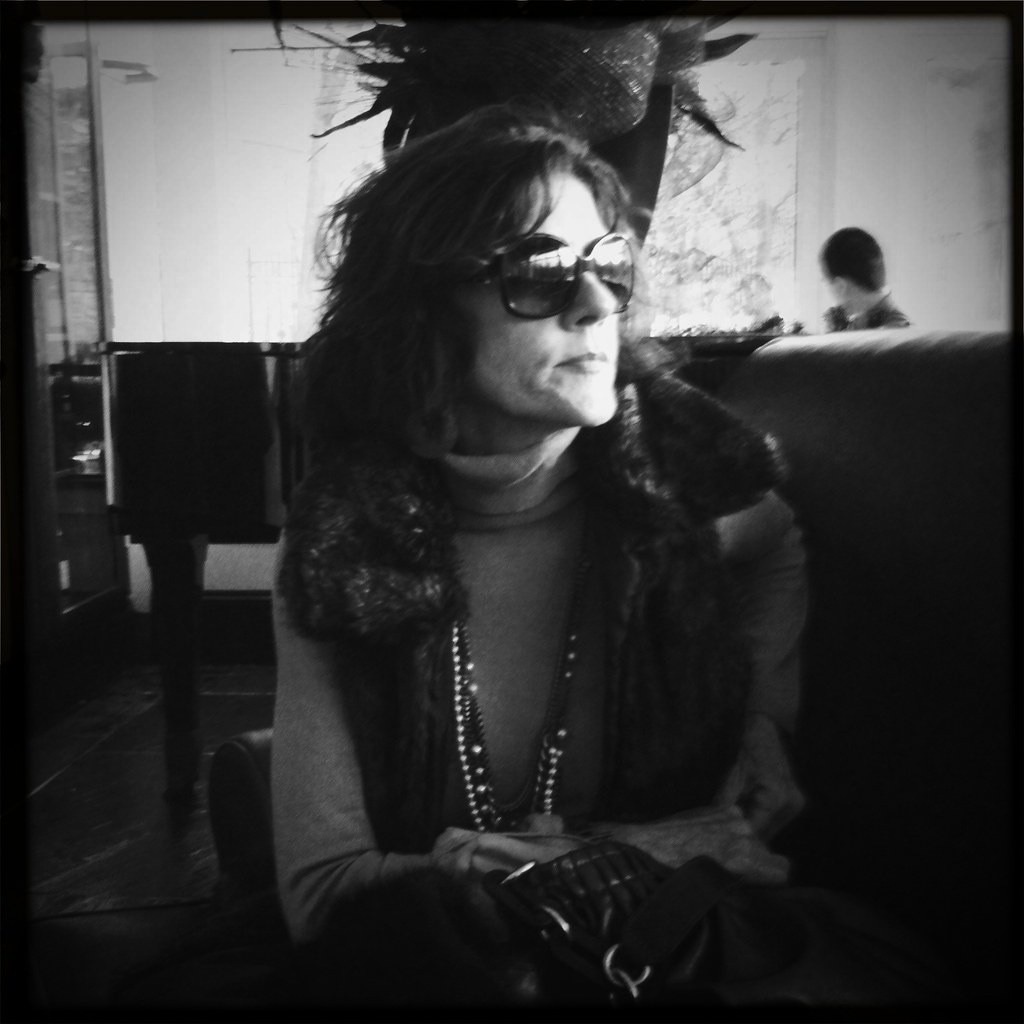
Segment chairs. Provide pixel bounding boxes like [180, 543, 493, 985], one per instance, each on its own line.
[207, 334, 1023, 1023]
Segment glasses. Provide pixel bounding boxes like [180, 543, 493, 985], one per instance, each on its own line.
[455, 234, 637, 321]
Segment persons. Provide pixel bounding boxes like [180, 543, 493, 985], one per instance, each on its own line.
[270, 102, 810, 1024]
[820, 229, 910, 333]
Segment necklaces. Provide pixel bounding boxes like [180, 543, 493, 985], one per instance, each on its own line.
[445, 598, 580, 835]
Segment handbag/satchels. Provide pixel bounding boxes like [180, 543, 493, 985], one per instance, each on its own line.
[490, 838, 945, 1024]
